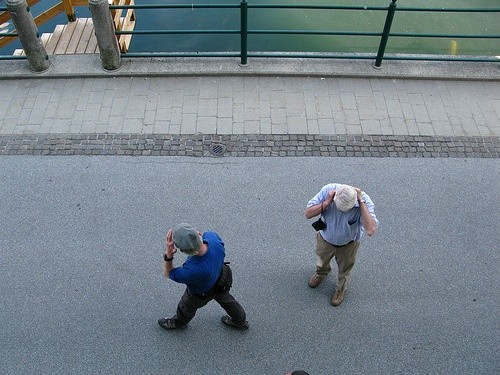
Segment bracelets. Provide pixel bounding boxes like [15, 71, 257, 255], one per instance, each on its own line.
[359, 199, 366, 203]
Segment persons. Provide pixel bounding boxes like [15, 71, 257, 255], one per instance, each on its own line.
[158, 223, 248, 331]
[305, 183, 379, 305]
[287, 370, 309, 375]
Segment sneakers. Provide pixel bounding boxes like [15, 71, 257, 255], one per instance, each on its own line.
[331, 290, 344, 306]
[159, 317, 186, 330]
[221, 315, 249, 329]
[308, 274, 324, 287]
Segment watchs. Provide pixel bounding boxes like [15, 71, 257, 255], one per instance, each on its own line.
[162, 254, 174, 261]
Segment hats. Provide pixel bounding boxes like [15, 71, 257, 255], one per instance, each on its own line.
[172, 223, 198, 250]
